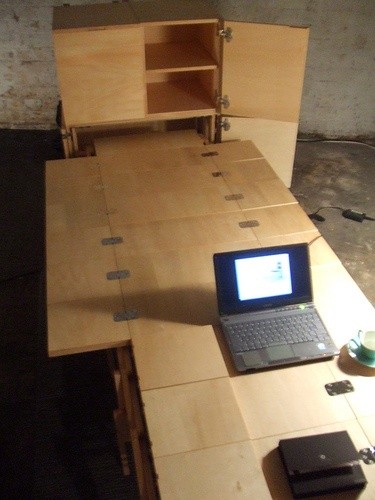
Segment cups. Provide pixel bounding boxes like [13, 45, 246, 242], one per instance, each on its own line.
[358, 330, 375, 359]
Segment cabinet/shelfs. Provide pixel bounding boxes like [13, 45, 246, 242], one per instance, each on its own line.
[44, 4, 375, 500]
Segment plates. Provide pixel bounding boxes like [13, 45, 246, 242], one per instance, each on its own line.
[346, 338, 375, 368]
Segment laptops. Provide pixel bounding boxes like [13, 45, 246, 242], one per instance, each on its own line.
[213, 242, 341, 373]
